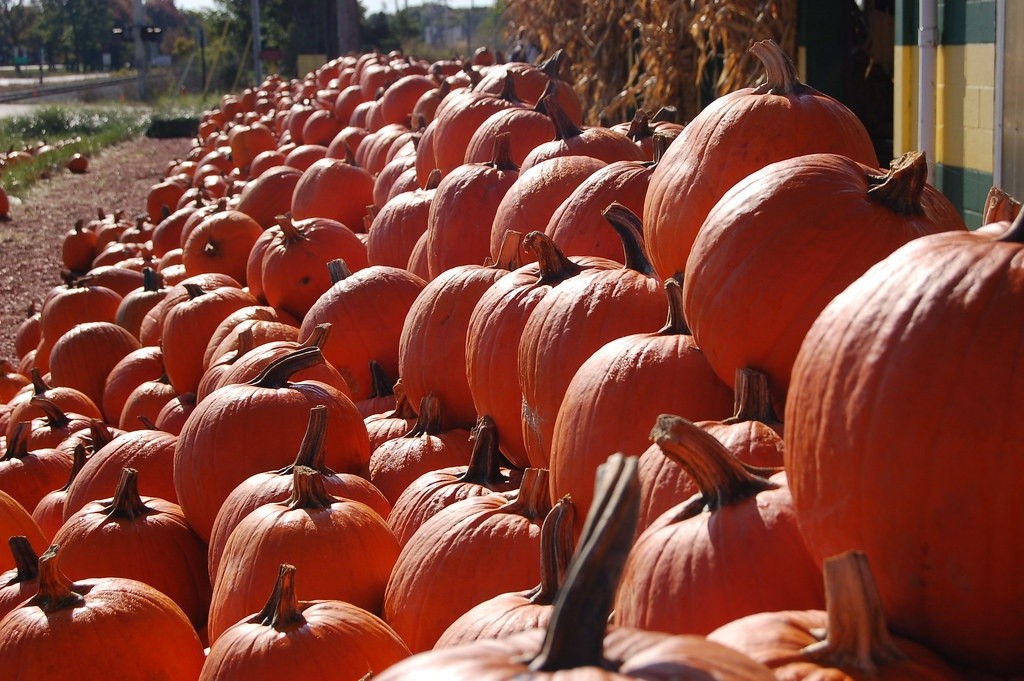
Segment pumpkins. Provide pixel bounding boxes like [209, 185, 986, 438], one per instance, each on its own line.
[0, 43, 1024, 681]
